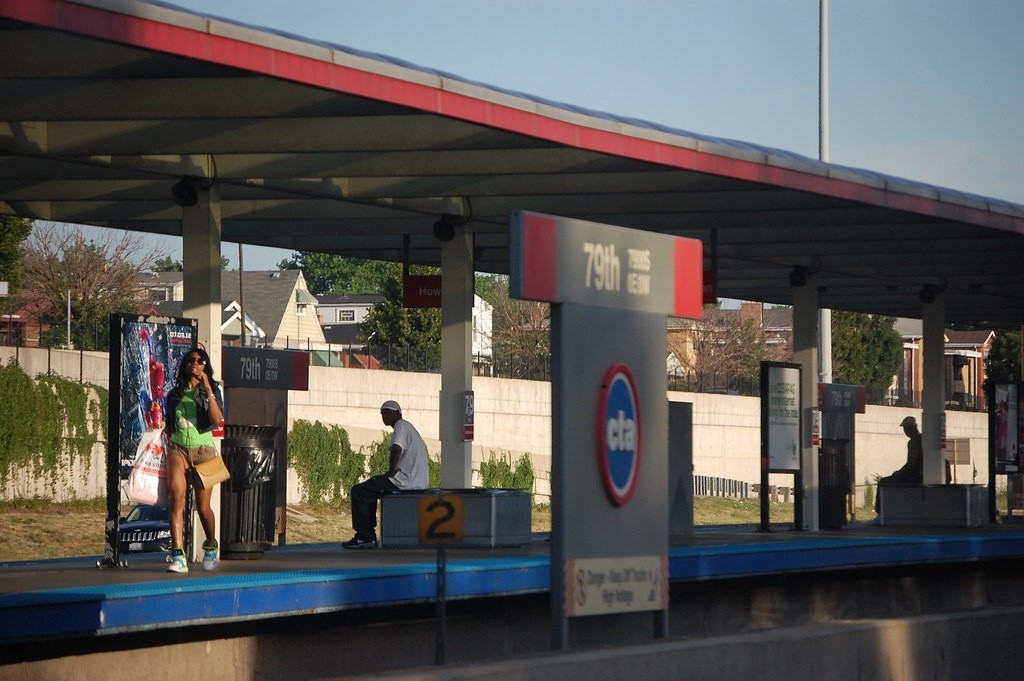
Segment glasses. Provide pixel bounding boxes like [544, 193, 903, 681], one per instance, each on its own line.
[185, 357, 206, 366]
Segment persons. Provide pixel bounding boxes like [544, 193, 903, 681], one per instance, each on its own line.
[163, 348, 225, 573]
[871, 416, 923, 523]
[342, 401, 431, 548]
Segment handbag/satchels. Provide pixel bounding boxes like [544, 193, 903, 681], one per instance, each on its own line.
[128, 430, 170, 507]
[192, 456, 231, 490]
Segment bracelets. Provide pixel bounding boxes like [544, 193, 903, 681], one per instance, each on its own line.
[206, 393, 217, 402]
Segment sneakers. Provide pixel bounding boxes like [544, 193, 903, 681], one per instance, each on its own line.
[202, 539, 219, 570]
[342, 536, 379, 548]
[168, 548, 189, 573]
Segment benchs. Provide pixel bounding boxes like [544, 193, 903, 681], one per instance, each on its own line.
[879, 482, 989, 526]
[378, 486, 534, 548]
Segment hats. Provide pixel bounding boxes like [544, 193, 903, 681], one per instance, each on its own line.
[900, 417, 916, 426]
[382, 400, 403, 414]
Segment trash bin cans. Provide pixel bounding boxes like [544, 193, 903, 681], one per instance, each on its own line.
[818, 438, 851, 530]
[220, 423, 283, 560]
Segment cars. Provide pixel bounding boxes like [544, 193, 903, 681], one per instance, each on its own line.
[119, 504, 173, 551]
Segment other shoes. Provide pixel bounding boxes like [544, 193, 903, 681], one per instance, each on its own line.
[864, 515, 881, 525]
